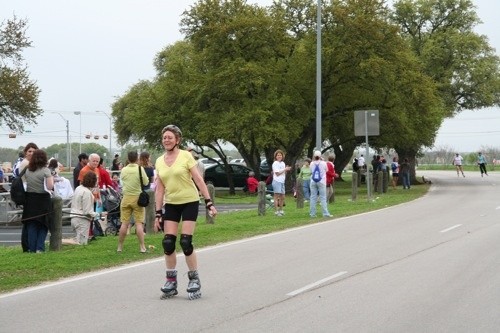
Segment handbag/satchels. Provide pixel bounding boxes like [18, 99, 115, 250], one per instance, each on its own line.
[137, 192, 150, 207]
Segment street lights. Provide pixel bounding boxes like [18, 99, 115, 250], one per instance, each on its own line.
[364, 111, 376, 196]
[51, 111, 69, 169]
[73, 111, 82, 154]
[96, 110, 111, 152]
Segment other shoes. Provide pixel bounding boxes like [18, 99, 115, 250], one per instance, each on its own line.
[275, 211, 282, 216]
[280, 212, 285, 215]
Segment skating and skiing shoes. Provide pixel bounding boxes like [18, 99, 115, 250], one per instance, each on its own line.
[186, 270, 202, 299]
[160, 270, 178, 300]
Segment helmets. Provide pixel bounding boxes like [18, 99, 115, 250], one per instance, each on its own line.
[161, 124, 182, 144]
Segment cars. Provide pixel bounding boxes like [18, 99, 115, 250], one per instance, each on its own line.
[197, 155, 272, 187]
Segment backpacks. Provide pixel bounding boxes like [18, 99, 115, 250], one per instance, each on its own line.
[10, 165, 28, 205]
[311, 162, 321, 182]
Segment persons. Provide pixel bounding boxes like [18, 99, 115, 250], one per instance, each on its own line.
[153, 124, 217, 299]
[452, 153, 465, 177]
[246, 172, 259, 193]
[14, 143, 165, 253]
[272, 150, 291, 216]
[475, 152, 489, 177]
[309, 151, 334, 218]
[193, 154, 205, 198]
[295, 163, 301, 177]
[326, 155, 339, 204]
[264, 170, 273, 191]
[352, 154, 411, 190]
[297, 158, 312, 203]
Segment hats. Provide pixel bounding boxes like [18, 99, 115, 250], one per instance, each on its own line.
[315, 151, 321, 157]
